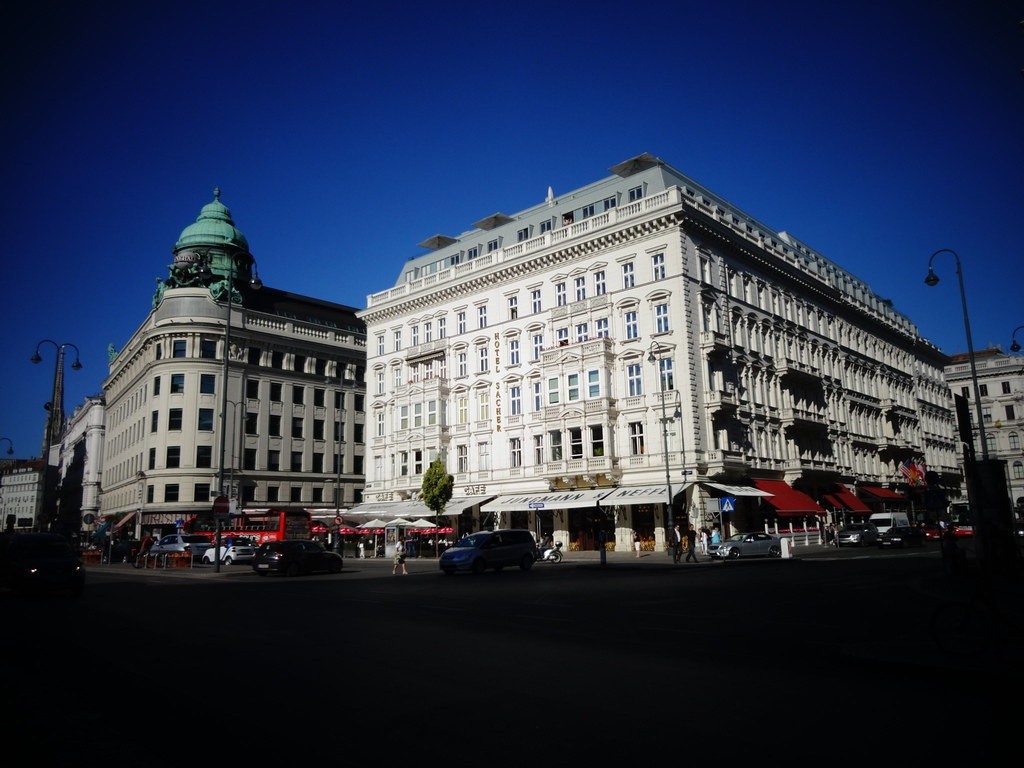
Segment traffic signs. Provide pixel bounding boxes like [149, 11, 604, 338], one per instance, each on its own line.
[528, 502, 545, 508]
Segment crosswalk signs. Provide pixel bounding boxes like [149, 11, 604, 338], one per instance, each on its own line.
[722, 497, 735, 512]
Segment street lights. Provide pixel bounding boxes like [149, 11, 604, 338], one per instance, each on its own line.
[221, 400, 251, 511]
[923, 247, 993, 462]
[213, 249, 262, 577]
[29, 339, 83, 469]
[644, 343, 680, 557]
[324, 363, 360, 554]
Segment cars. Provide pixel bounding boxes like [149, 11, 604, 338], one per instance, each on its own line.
[0, 532, 86, 598]
[919, 524, 947, 542]
[834, 523, 878, 549]
[203, 536, 258, 567]
[877, 526, 928, 549]
[148, 532, 210, 563]
[708, 530, 782, 560]
[438, 528, 539, 575]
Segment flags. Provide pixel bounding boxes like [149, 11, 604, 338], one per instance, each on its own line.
[903, 460, 928, 486]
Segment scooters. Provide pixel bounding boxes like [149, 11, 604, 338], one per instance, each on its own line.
[535, 541, 565, 564]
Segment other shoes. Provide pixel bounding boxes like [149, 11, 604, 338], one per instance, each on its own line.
[695, 561, 699, 563]
[402, 572, 408, 575]
[685, 560, 690, 562]
[392, 570, 395, 575]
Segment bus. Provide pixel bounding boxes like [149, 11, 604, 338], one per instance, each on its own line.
[946, 498, 974, 538]
[182, 507, 312, 552]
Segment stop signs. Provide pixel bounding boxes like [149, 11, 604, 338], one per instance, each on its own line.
[214, 495, 229, 514]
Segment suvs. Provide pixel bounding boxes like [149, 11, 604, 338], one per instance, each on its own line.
[252, 539, 344, 578]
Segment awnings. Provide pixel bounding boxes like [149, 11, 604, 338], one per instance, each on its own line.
[705, 482, 774, 496]
[480, 488, 615, 512]
[598, 482, 693, 505]
[115, 512, 136, 528]
[347, 502, 401, 516]
[856, 485, 906, 499]
[819, 483, 871, 513]
[754, 480, 826, 516]
[386, 495, 496, 518]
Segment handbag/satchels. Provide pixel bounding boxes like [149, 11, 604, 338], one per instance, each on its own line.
[669, 539, 674, 548]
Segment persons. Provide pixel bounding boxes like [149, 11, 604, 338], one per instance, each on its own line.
[392, 535, 408, 574]
[826, 523, 836, 544]
[633, 532, 641, 558]
[357, 534, 369, 558]
[539, 532, 550, 559]
[668, 523, 721, 564]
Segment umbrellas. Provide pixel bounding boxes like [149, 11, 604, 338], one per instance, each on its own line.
[311, 525, 328, 540]
[359, 528, 384, 534]
[331, 525, 358, 540]
[386, 517, 413, 537]
[356, 519, 386, 557]
[409, 519, 455, 556]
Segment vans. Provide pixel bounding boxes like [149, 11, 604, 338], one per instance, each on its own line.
[869, 512, 911, 545]
